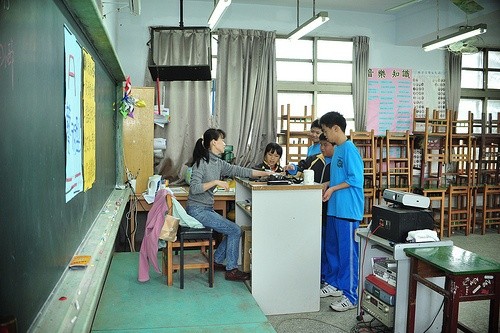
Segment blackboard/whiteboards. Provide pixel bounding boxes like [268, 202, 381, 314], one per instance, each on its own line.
[0, 0, 123, 333]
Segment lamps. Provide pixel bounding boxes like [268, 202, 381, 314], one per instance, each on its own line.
[422, 0, 487, 51]
[287, 0, 329, 42]
[207, 0, 231, 31]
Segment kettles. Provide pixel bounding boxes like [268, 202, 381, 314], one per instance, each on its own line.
[147, 174, 161, 195]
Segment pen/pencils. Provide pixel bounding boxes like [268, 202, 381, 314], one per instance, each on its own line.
[125, 168, 140, 179]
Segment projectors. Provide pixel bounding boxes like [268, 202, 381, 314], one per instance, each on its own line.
[383, 189, 430, 209]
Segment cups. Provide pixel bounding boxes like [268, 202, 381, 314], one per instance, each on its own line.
[304, 170, 314, 185]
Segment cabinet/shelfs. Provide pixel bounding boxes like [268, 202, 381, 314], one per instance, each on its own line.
[352, 228, 454, 333]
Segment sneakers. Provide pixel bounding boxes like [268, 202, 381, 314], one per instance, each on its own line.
[320, 285, 343, 298]
[330, 297, 358, 312]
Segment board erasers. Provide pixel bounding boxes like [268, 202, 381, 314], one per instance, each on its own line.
[69, 255, 92, 270]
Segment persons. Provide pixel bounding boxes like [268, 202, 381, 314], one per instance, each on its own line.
[286, 111, 365, 312]
[184, 128, 286, 282]
[250, 142, 292, 177]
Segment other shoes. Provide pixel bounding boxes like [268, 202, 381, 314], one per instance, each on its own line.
[214, 262, 225, 271]
[225, 268, 251, 280]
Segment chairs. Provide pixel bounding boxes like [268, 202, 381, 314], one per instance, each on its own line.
[157, 191, 217, 289]
[280, 105, 500, 238]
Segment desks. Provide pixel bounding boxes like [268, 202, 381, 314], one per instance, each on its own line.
[403, 246, 500, 333]
[130, 194, 235, 255]
[235, 176, 323, 316]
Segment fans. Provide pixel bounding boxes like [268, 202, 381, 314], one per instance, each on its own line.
[448, 24, 484, 56]
